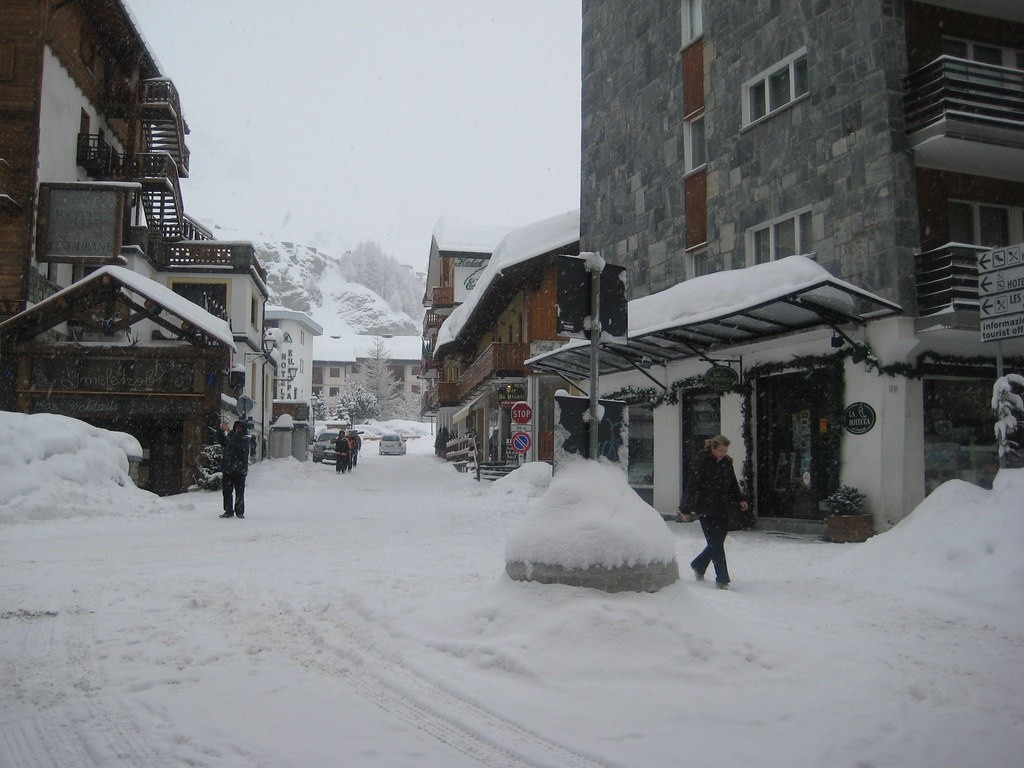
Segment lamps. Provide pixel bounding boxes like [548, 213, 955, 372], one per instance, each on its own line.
[641, 354, 653, 369]
[831, 330, 845, 348]
[273, 363, 298, 381]
[245, 330, 277, 362]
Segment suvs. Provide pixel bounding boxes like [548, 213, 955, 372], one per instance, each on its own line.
[311, 429, 349, 463]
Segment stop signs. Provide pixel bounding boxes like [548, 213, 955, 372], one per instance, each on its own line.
[511, 401, 532, 423]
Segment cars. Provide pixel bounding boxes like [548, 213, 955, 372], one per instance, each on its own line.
[379, 434, 407, 455]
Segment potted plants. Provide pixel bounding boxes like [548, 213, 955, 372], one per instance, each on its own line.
[824, 485, 873, 542]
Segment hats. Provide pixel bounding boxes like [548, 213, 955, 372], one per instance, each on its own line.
[234, 421, 249, 435]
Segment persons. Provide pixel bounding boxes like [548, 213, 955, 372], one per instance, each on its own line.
[335, 430, 361, 473]
[680, 435, 748, 589]
[217, 420, 250, 519]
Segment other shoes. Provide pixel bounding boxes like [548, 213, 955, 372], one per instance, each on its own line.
[715, 581, 732, 590]
[220, 511, 235, 518]
[236, 513, 244, 518]
[690, 562, 705, 582]
